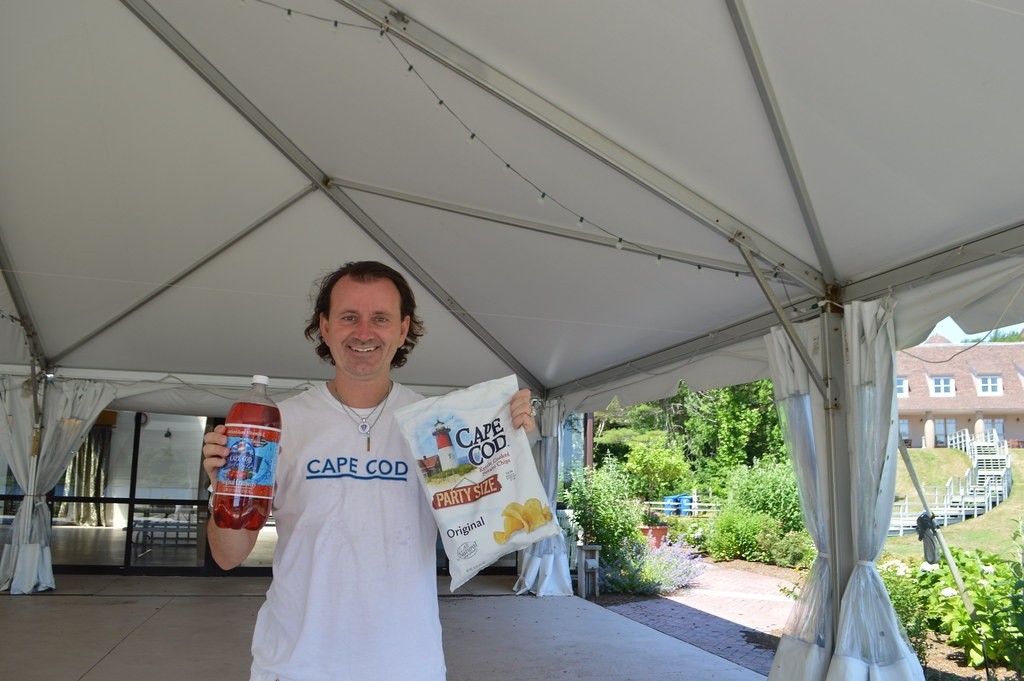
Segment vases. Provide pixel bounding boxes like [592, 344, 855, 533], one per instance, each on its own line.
[638, 527, 668, 549]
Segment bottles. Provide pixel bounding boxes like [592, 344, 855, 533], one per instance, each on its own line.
[213, 374, 282, 531]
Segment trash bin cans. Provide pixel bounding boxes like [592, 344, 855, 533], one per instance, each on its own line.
[662, 495, 700, 517]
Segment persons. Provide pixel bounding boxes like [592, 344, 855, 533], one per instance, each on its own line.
[204, 261, 538, 679]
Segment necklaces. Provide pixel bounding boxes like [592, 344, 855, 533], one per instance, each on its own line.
[331, 377, 390, 452]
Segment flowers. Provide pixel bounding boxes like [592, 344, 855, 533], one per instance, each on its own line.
[642, 508, 667, 526]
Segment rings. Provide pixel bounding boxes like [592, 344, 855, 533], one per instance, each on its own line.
[530, 404, 536, 417]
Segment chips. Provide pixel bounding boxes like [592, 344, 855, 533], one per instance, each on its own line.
[493, 499, 552, 544]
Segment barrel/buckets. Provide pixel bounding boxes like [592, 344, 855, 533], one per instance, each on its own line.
[663, 493, 692, 517]
